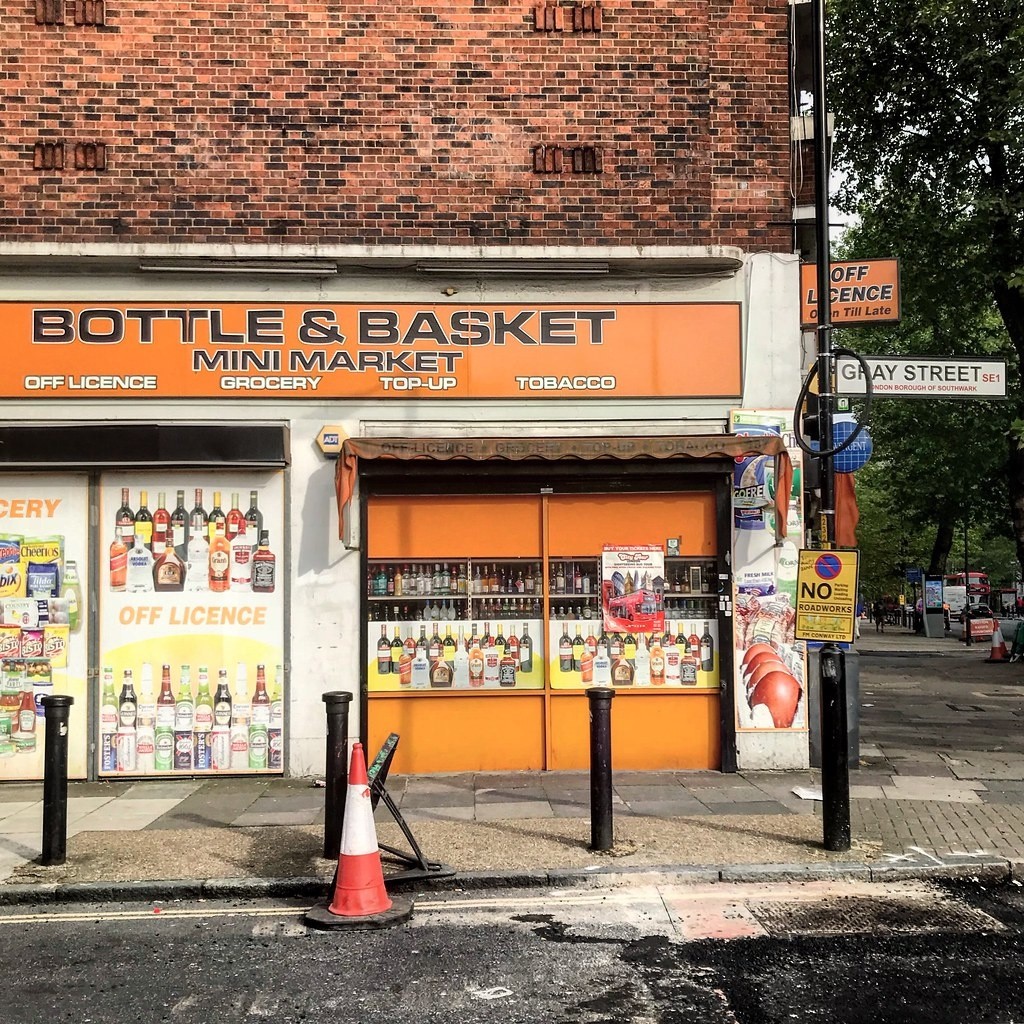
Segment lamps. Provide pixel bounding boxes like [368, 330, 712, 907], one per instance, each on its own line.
[415, 260, 610, 274]
[139, 257, 338, 274]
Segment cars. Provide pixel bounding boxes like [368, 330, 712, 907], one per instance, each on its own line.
[959, 602, 993, 624]
[883, 594, 914, 617]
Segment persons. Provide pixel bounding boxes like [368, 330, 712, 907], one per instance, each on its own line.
[869, 597, 902, 633]
[854, 603, 863, 639]
[1017, 597, 1024, 618]
[1003, 601, 1010, 617]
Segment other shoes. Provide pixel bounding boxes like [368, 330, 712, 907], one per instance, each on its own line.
[881, 630, 884, 633]
[857, 636, 859, 639]
[876, 629, 878, 632]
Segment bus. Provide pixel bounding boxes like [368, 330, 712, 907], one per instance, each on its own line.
[944, 573, 991, 618]
[608, 589, 657, 622]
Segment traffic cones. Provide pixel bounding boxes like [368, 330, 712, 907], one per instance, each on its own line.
[303, 743, 414, 931]
[984, 618, 1013, 663]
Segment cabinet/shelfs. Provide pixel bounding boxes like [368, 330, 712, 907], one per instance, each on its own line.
[364, 557, 718, 620]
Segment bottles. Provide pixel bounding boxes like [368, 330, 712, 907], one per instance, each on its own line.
[0, 680, 37, 736]
[378, 623, 533, 688]
[366, 564, 598, 620]
[59, 560, 83, 633]
[663, 564, 717, 618]
[108, 488, 276, 593]
[99, 662, 284, 729]
[559, 622, 714, 686]
